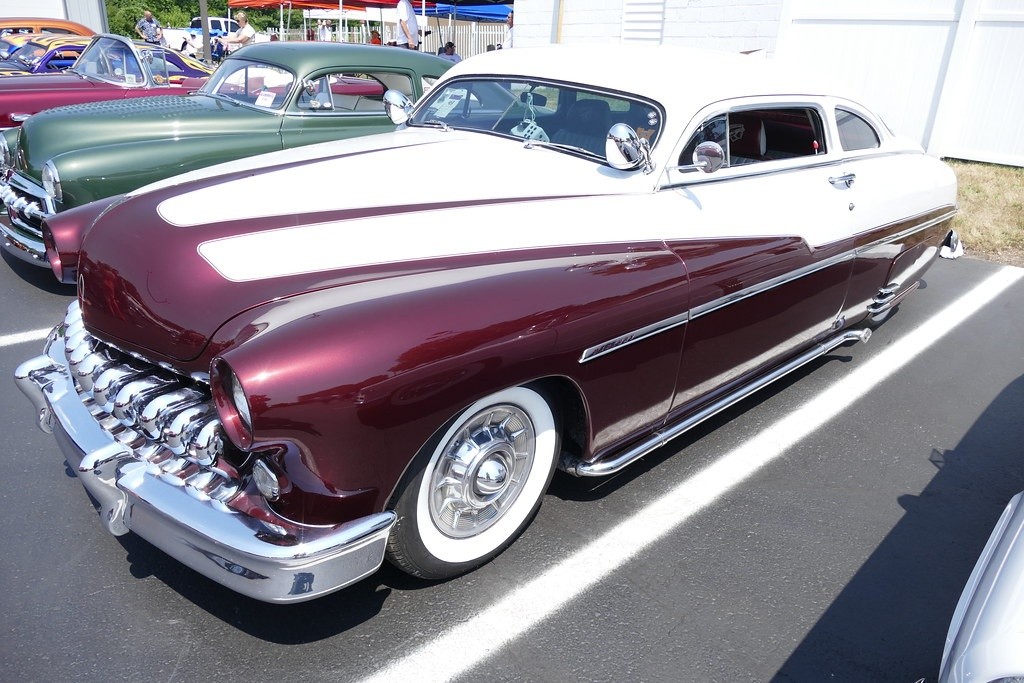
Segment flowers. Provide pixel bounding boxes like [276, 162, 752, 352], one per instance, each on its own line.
[310, 100, 332, 108]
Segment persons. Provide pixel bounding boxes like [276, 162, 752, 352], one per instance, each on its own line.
[396, 0, 422, 51]
[487, 44, 496, 52]
[501, 10, 514, 49]
[135, 11, 163, 46]
[367, 30, 381, 44]
[214, 13, 256, 49]
[317, 19, 333, 41]
[439, 42, 463, 63]
[180, 30, 229, 64]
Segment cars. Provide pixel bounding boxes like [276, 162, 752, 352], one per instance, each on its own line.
[0, 16, 218, 83]
[15, 39, 959, 606]
[0, 33, 388, 133]
[0, 42, 557, 270]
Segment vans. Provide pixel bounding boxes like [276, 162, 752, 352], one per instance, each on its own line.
[189, 16, 241, 36]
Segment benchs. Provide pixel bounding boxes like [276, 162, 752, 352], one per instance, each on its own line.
[315, 92, 386, 111]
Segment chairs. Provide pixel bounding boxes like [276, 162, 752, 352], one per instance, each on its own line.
[550, 99, 613, 157]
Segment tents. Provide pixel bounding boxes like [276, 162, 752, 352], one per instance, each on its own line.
[227, 0, 514, 57]
